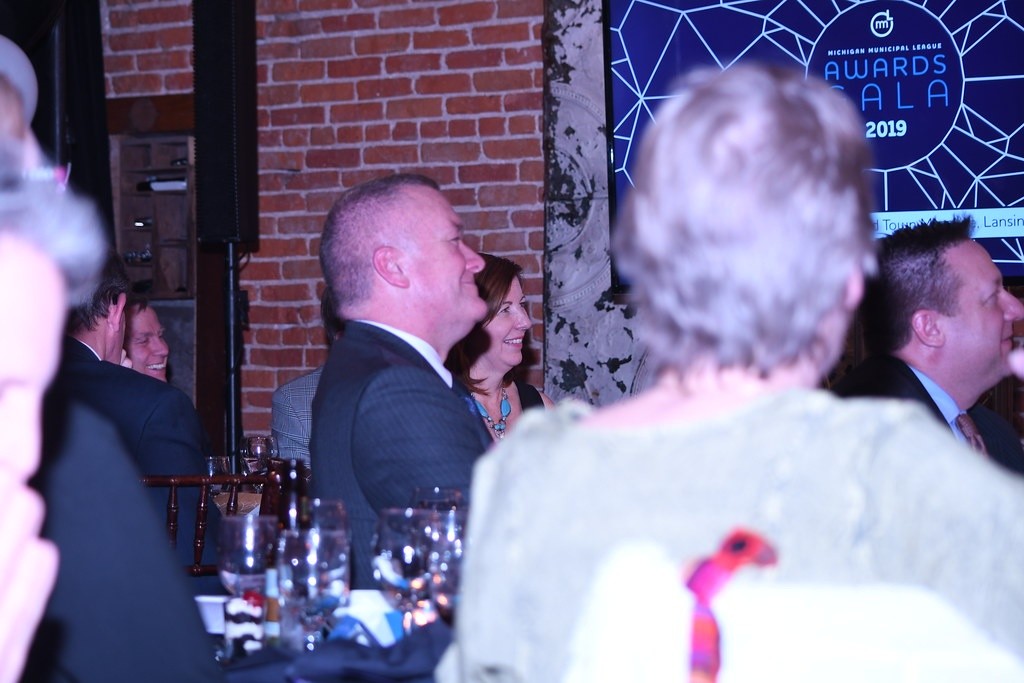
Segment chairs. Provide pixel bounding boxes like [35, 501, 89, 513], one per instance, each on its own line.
[142, 473, 281, 592]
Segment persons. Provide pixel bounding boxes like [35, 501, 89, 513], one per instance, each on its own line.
[0, 136, 114, 682]
[121, 287, 170, 386]
[440, 253, 554, 453]
[270, 285, 348, 477]
[1, 34, 69, 204]
[38, 248, 237, 602]
[824, 214, 1022, 478]
[308, 174, 500, 597]
[435, 66, 1023, 683]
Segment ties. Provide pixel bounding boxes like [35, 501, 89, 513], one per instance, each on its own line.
[453, 374, 494, 450]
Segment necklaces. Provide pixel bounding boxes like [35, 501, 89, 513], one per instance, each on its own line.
[461, 381, 511, 440]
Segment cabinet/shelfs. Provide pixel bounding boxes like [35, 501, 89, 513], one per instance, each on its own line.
[116, 136, 195, 301]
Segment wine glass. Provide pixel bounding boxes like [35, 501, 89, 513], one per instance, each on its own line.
[192, 432, 469, 660]
